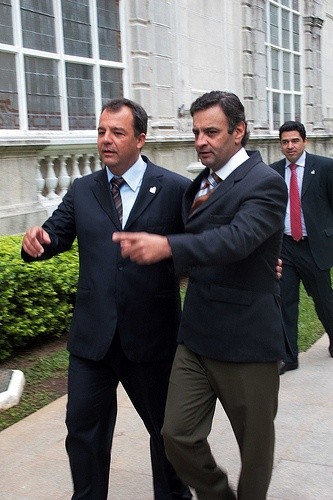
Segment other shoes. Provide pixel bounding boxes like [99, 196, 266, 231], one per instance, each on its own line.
[278, 359, 298, 375]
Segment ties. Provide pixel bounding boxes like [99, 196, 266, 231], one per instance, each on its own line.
[189, 172, 223, 216]
[288, 164, 302, 242]
[109, 176, 127, 228]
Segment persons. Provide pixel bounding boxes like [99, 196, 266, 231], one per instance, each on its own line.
[111, 90, 288, 500]
[267, 121, 333, 374]
[21, 96, 282, 500]
